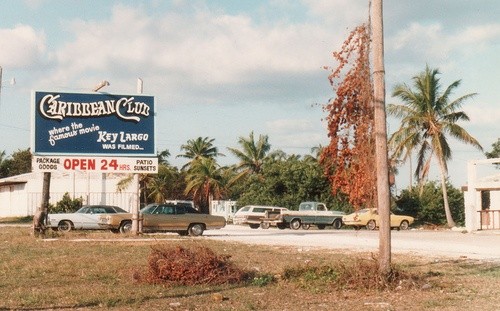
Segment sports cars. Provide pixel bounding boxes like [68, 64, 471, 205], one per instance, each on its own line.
[342, 208, 414, 231]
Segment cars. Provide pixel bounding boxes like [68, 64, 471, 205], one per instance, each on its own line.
[47, 205, 132, 233]
[139, 204, 226, 236]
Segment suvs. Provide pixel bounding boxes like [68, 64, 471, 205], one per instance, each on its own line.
[233, 205, 288, 229]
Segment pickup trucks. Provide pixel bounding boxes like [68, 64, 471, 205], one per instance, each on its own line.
[264, 202, 346, 230]
